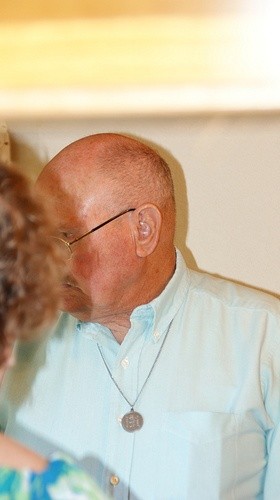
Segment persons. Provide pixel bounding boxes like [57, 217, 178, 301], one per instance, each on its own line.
[0, 161, 114, 500]
[0, 133, 280, 500]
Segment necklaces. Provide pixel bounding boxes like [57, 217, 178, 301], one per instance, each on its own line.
[96, 321, 173, 433]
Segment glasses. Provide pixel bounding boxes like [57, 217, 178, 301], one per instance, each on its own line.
[48, 208, 137, 261]
